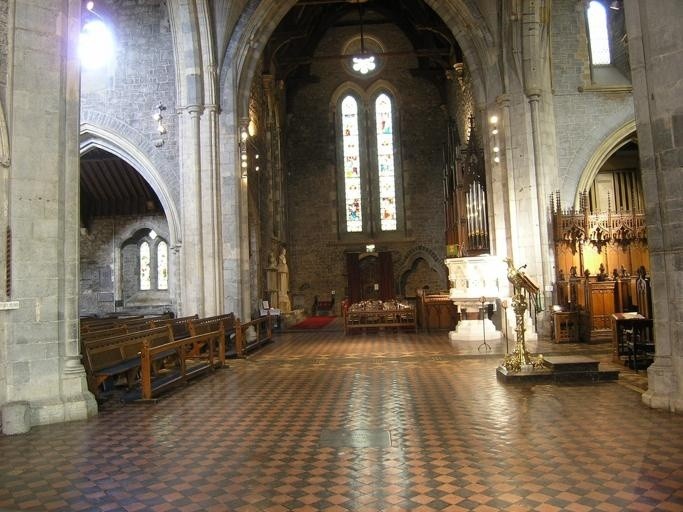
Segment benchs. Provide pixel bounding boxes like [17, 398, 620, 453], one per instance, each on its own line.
[79, 313, 246, 404]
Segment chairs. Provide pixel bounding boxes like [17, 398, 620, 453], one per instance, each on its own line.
[341, 296, 417, 337]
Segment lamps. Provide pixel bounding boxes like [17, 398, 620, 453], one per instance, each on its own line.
[346, 3, 378, 76]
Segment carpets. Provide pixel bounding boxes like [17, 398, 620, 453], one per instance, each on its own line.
[289, 315, 338, 330]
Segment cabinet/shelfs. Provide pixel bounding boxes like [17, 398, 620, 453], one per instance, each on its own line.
[612, 309, 655, 374]
[416, 291, 454, 331]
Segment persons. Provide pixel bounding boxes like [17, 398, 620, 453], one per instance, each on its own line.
[277, 247, 285, 263]
